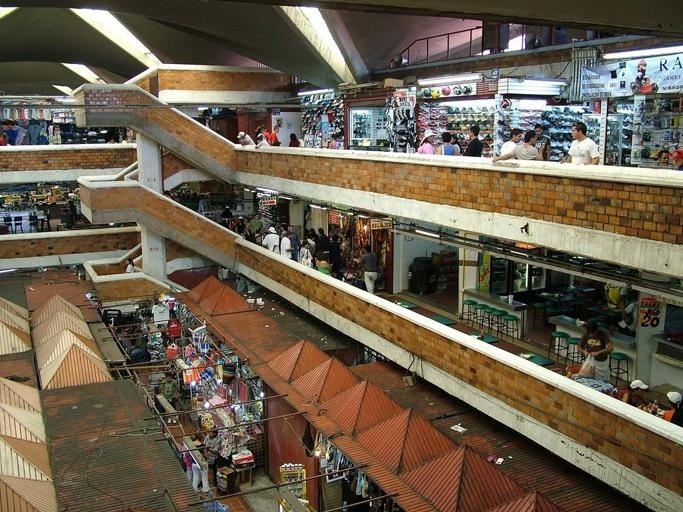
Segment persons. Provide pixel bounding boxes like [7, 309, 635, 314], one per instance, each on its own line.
[417, 123, 601, 165]
[126, 257, 135, 273]
[577, 283, 683, 427]
[635, 63, 646, 95]
[391, 54, 408, 68]
[213, 204, 378, 294]
[184, 428, 223, 493]
[0, 132, 11, 146]
[526, 32, 545, 48]
[237, 124, 301, 148]
[657, 149, 683, 170]
[127, 338, 180, 424]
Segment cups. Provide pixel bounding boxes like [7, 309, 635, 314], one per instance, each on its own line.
[509, 295, 513, 305]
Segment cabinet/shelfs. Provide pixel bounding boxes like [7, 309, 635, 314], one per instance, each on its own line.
[279, 465, 307, 512]
[216, 467, 235, 495]
[350, 101, 673, 169]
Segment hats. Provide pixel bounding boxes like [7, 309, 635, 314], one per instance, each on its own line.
[269, 227, 277, 233]
[237, 132, 245, 140]
[667, 392, 682, 404]
[630, 379, 648, 390]
[425, 130, 436, 139]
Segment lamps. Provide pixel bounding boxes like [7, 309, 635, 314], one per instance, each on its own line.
[308, 203, 328, 211]
[279, 195, 296, 201]
[510, 251, 527, 257]
[415, 229, 440, 238]
[256, 187, 279, 195]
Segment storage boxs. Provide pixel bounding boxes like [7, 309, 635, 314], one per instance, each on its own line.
[652, 334, 683, 361]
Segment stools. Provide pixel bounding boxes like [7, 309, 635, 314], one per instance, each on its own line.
[652, 383, 683, 404]
[460, 297, 629, 390]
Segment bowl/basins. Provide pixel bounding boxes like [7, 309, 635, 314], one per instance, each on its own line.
[499, 296, 507, 300]
[576, 321, 586, 326]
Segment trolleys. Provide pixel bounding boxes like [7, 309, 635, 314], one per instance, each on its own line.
[344, 257, 366, 289]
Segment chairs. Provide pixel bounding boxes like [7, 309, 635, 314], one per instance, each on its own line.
[14, 216, 23, 234]
[4, 217, 13, 234]
[319, 268, 333, 277]
[1, 194, 29, 213]
[29, 190, 81, 233]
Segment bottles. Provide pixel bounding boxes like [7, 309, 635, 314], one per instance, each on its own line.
[652, 400, 658, 416]
